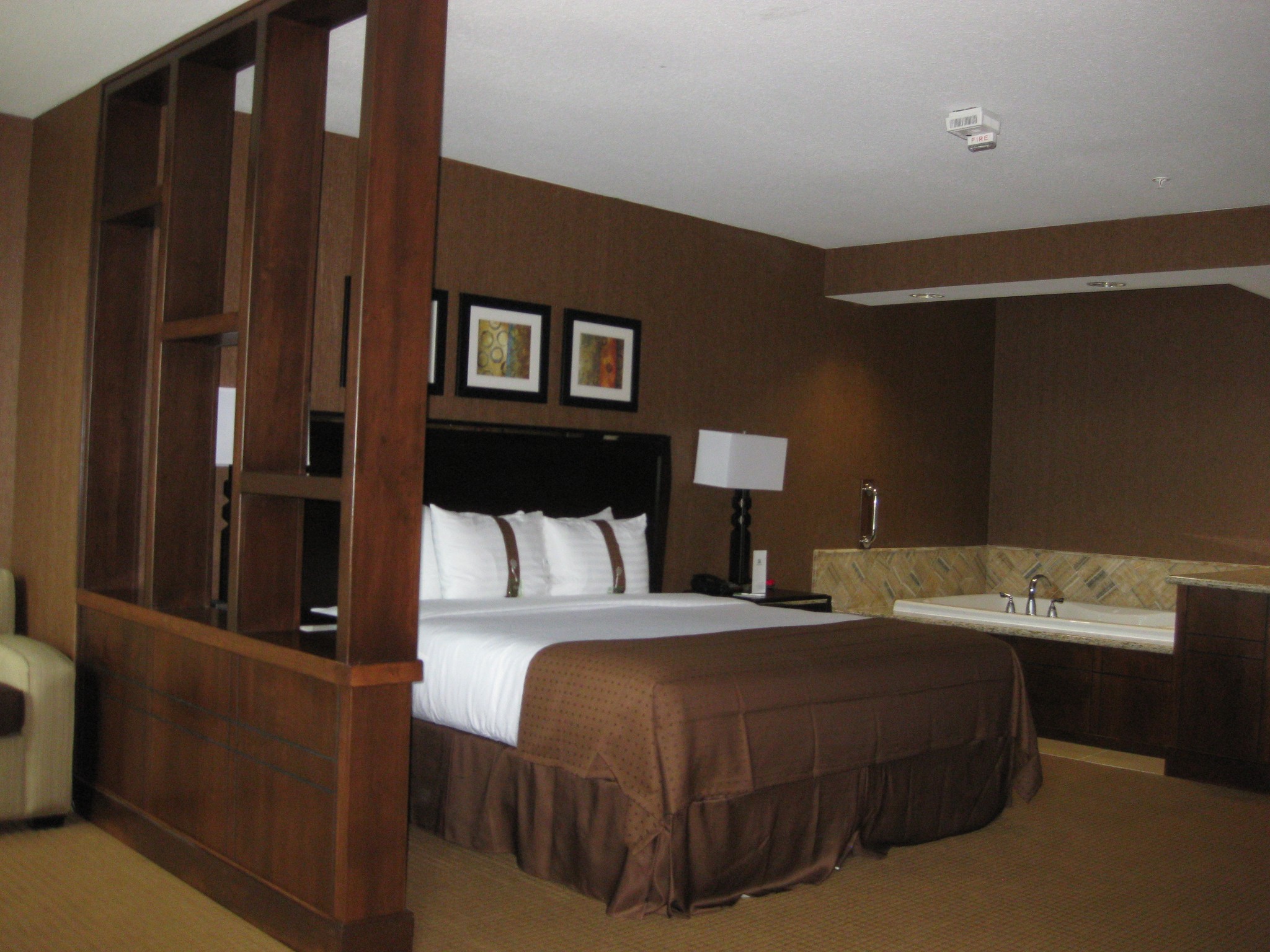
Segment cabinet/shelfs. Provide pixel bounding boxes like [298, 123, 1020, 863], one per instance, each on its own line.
[73, 0, 453, 668]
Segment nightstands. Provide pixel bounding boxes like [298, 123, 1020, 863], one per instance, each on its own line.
[724, 586, 833, 613]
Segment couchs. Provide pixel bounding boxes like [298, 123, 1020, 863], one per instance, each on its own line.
[0, 568, 78, 829]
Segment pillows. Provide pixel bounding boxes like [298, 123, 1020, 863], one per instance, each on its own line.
[422, 501, 652, 601]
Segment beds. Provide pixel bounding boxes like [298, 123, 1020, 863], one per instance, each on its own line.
[300, 410, 1047, 922]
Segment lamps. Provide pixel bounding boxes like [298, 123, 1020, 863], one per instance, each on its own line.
[693, 428, 787, 591]
[216, 387, 311, 598]
[943, 108, 998, 152]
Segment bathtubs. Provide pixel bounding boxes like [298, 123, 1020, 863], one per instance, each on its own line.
[893, 593, 1176, 749]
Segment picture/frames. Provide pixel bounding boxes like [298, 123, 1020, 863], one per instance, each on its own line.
[559, 308, 642, 412]
[454, 291, 550, 404]
[338, 276, 450, 396]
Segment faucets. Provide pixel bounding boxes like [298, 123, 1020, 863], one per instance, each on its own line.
[1026, 574, 1052, 616]
